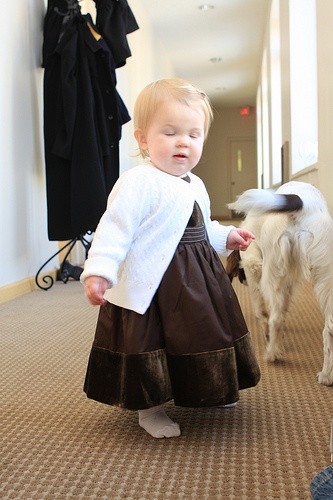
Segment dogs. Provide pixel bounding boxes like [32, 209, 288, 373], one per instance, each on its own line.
[227, 179, 332, 386]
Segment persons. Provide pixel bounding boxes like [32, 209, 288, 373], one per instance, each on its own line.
[80, 77, 261, 439]
